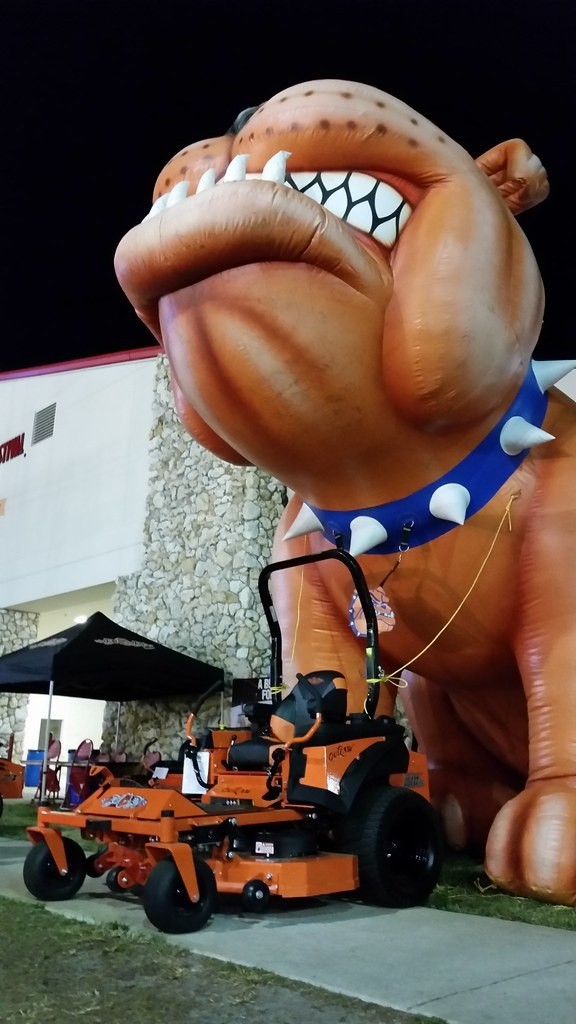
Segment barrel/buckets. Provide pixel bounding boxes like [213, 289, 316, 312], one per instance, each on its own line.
[24, 750, 44, 787]
[66, 748, 99, 804]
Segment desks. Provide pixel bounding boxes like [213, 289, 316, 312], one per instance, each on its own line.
[19, 760, 140, 801]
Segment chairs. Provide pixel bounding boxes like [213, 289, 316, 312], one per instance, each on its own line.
[219, 669, 348, 771]
[143, 751, 160, 772]
[59, 738, 93, 811]
[31, 739, 61, 805]
[112, 751, 128, 762]
[95, 751, 110, 762]
